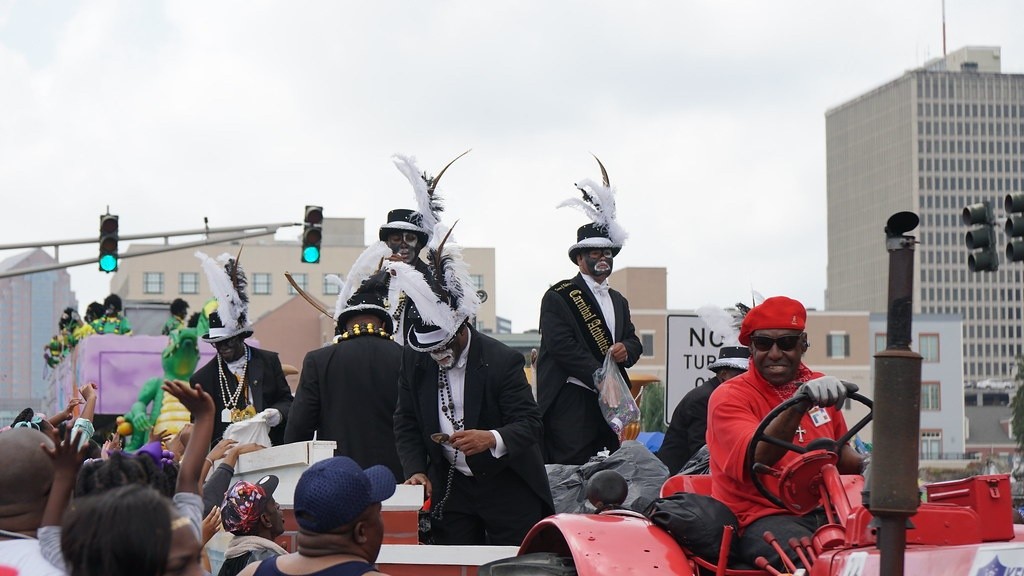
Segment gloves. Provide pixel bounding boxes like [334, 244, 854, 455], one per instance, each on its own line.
[793, 376, 859, 411]
[262, 408, 283, 427]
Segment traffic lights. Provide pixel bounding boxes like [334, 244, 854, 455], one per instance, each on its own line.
[98, 214, 121, 274]
[1001, 189, 1024, 261]
[962, 198, 1002, 274]
[300, 206, 325, 264]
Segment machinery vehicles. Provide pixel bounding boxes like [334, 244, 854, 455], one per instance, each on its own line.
[474, 208, 1023, 576]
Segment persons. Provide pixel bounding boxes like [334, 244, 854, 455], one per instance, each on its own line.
[706, 297, 868, 576]
[44, 293, 132, 369]
[346, 209, 443, 346]
[236, 456, 396, 576]
[0, 381, 268, 576]
[538, 222, 643, 465]
[393, 302, 556, 547]
[190, 312, 292, 454]
[657, 346, 751, 477]
[283, 293, 403, 470]
[217, 474, 290, 576]
[161, 298, 190, 337]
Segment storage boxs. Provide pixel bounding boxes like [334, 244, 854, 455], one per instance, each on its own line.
[924, 475, 1015, 543]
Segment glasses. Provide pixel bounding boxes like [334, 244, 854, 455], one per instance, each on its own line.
[211, 336, 241, 348]
[751, 333, 803, 351]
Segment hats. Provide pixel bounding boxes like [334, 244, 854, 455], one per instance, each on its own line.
[557, 154, 625, 265]
[335, 256, 393, 337]
[738, 296, 806, 346]
[294, 455, 396, 531]
[194, 243, 253, 342]
[387, 220, 481, 352]
[697, 302, 750, 373]
[221, 475, 280, 536]
[379, 148, 471, 248]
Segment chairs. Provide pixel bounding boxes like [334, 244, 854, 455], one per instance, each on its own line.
[659, 475, 772, 576]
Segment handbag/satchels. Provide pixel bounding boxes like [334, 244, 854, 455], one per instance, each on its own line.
[642, 491, 740, 567]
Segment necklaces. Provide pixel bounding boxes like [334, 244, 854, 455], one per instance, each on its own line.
[100, 315, 120, 334]
[218, 344, 248, 422]
[431, 369, 464, 520]
[394, 293, 406, 335]
[334, 322, 394, 345]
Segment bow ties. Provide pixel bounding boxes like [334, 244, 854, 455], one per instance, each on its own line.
[593, 282, 610, 296]
[455, 359, 466, 368]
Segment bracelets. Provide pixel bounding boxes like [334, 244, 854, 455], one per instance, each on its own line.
[206, 458, 212, 464]
